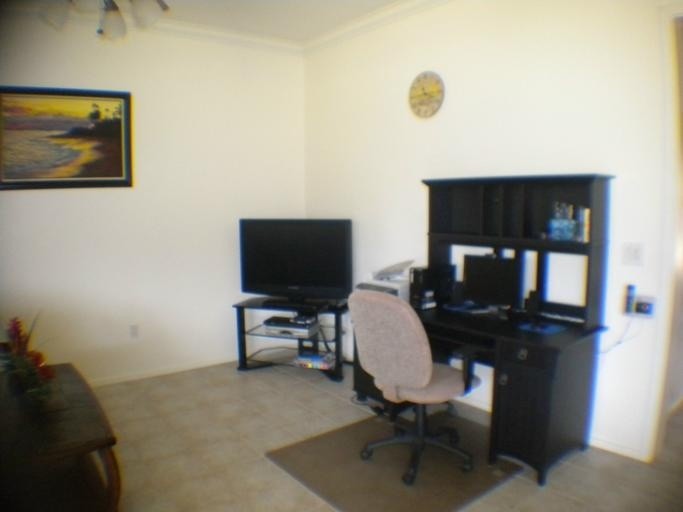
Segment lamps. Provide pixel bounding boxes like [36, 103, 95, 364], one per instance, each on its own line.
[44, 0, 176, 39]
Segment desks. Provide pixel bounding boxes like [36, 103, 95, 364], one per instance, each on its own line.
[351, 299, 608, 485]
[0, 363, 125, 511]
[234, 294, 353, 381]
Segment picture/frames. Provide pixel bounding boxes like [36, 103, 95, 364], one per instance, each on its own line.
[0, 83, 134, 188]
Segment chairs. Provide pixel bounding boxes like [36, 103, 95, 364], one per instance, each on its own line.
[349, 290, 480, 486]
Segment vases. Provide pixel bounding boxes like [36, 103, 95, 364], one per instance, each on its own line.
[8, 367, 27, 391]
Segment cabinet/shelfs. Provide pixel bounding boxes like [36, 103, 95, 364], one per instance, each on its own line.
[418, 172, 612, 336]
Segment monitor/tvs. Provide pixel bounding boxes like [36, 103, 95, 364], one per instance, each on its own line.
[239, 218, 352, 306]
[463, 255, 517, 306]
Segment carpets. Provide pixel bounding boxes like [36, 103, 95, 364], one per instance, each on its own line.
[265, 409, 529, 512]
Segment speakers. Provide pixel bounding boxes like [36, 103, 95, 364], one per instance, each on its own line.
[526, 290, 539, 318]
[450, 281, 464, 308]
[353, 282, 398, 407]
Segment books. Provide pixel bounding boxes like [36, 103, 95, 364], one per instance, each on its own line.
[294, 350, 336, 372]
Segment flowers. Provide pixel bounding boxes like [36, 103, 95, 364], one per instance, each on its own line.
[0, 312, 55, 394]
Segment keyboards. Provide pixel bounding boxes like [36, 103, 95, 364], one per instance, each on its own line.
[436, 308, 488, 329]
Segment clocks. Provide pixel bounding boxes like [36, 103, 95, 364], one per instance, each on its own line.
[409, 70, 445, 119]
